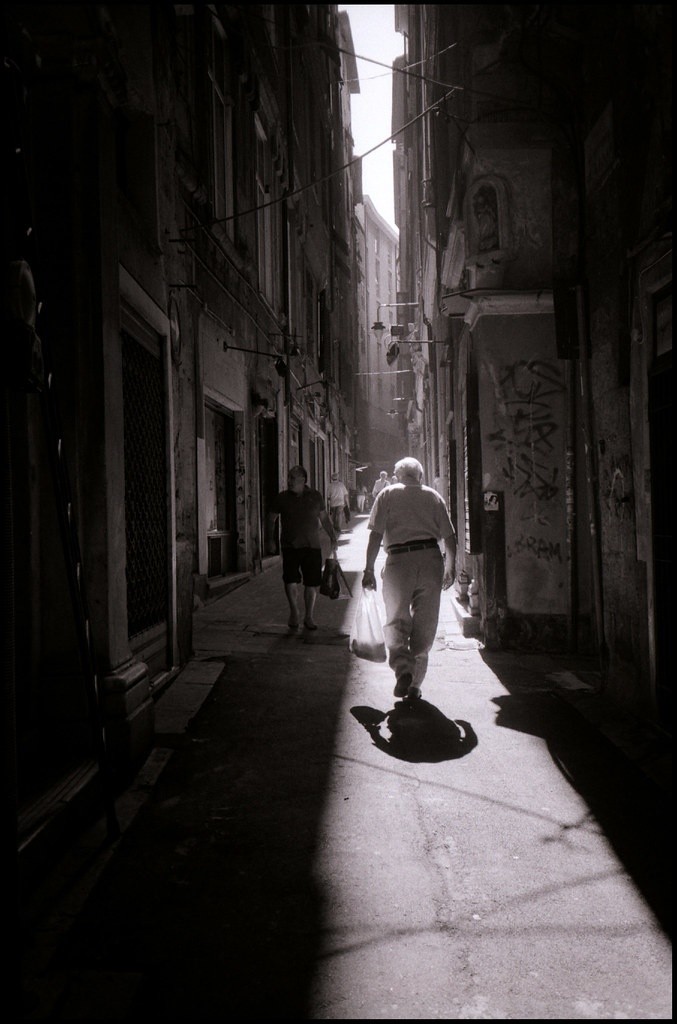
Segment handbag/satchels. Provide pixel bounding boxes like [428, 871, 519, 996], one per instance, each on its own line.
[319, 550, 340, 600]
[349, 584, 386, 663]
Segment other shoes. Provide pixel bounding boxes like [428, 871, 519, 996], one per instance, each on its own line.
[304, 618, 318, 631]
[287, 618, 298, 631]
[394, 673, 411, 697]
[408, 687, 422, 700]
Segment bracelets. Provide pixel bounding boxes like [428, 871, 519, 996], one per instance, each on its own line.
[363, 569, 374, 573]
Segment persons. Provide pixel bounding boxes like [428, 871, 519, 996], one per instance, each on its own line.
[361, 455, 457, 702]
[372, 470, 391, 498]
[327, 473, 350, 533]
[267, 465, 338, 630]
[391, 475, 398, 484]
[355, 481, 368, 513]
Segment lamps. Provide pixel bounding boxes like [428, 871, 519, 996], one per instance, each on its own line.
[223, 341, 289, 379]
[371, 302, 420, 339]
[268, 327, 305, 356]
[386, 340, 441, 369]
[297, 380, 328, 391]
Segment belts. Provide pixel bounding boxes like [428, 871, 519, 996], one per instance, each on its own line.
[387, 543, 439, 556]
